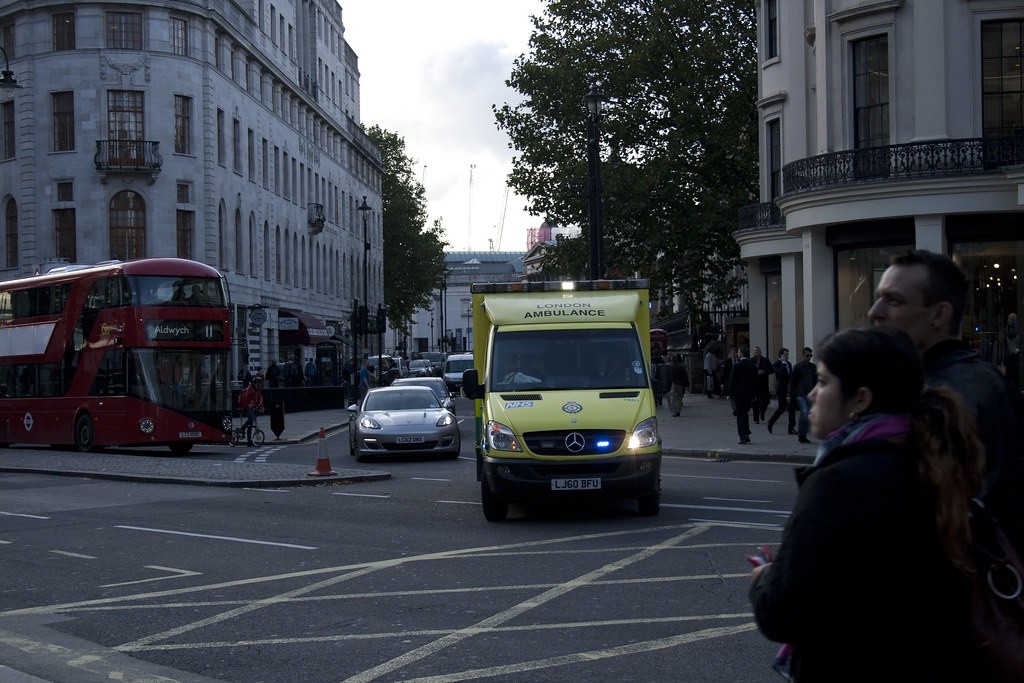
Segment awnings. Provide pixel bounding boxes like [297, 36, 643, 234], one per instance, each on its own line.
[332, 335, 353, 346]
[278, 310, 329, 346]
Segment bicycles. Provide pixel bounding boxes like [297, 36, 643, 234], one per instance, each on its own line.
[229, 405, 265, 447]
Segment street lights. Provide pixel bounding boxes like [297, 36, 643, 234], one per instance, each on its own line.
[582, 78, 607, 280]
[442, 268, 448, 352]
[357, 195, 373, 360]
[466, 306, 471, 351]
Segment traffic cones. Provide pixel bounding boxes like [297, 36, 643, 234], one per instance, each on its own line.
[306, 427, 338, 476]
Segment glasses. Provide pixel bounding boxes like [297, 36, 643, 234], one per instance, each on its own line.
[806, 354, 812, 358]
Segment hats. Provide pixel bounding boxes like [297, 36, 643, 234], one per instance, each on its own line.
[252, 376, 262, 385]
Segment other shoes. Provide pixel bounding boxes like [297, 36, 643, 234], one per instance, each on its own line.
[788, 428, 798, 434]
[738, 436, 751, 444]
[247, 442, 255, 447]
[798, 437, 811, 443]
[672, 412, 681, 417]
[767, 419, 773, 433]
[759, 412, 765, 421]
[240, 429, 245, 434]
[755, 420, 759, 424]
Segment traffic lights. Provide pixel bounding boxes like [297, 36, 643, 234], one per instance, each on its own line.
[357, 305, 370, 336]
[376, 309, 386, 333]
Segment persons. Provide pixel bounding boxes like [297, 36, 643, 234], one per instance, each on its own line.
[120, 368, 145, 394]
[305, 358, 317, 386]
[750, 346, 774, 424]
[868, 251, 1024, 567]
[786, 347, 818, 444]
[257, 367, 264, 388]
[238, 377, 265, 446]
[281, 359, 306, 387]
[721, 347, 752, 444]
[650, 350, 689, 416]
[768, 348, 797, 434]
[171, 285, 203, 301]
[703, 347, 724, 400]
[1003, 313, 1017, 347]
[749, 328, 1024, 682]
[507, 353, 543, 381]
[344, 359, 378, 399]
[239, 366, 252, 386]
[266, 360, 281, 388]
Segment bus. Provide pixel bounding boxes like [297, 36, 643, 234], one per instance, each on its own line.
[0, 258, 250, 452]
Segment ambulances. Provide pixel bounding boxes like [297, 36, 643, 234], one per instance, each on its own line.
[461, 276, 673, 522]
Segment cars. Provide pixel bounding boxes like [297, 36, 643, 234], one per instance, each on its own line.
[367, 351, 474, 416]
[348, 386, 461, 462]
